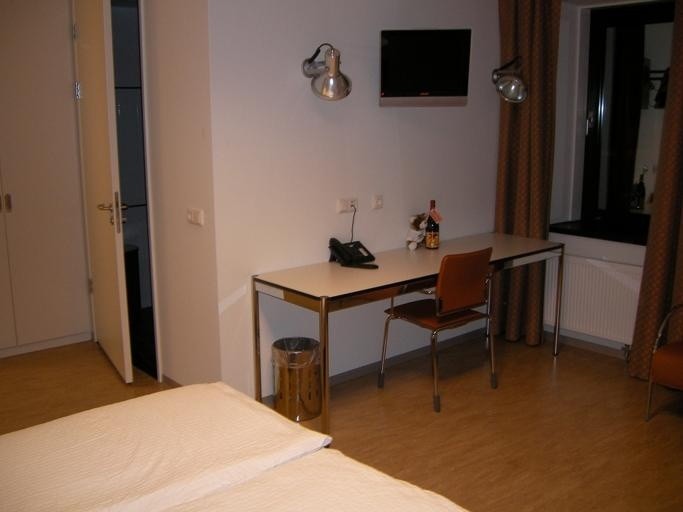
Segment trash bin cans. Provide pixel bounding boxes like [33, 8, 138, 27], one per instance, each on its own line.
[272, 337, 321, 422]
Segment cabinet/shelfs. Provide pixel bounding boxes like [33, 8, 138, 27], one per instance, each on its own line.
[1, 3, 96, 356]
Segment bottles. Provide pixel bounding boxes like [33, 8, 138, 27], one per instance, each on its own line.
[426, 199, 441, 250]
[637, 171, 647, 208]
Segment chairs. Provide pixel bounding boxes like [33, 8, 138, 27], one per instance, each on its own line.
[640, 301, 682, 421]
[377, 247, 497, 411]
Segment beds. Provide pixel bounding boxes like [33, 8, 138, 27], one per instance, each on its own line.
[0, 381, 333, 511]
[165, 447, 468, 511]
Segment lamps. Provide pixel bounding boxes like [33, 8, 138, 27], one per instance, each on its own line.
[492, 56, 527, 102]
[302, 45, 352, 100]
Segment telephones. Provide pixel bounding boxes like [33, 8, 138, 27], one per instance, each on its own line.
[328, 237, 379, 269]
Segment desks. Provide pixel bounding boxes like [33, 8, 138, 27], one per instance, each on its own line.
[251, 233, 565, 448]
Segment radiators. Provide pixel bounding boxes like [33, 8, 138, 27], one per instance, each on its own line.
[543, 232, 647, 350]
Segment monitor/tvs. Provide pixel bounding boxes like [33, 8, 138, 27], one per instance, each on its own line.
[380, 29, 470, 108]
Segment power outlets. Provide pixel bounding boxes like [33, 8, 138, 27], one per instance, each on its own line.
[348, 199, 358, 212]
